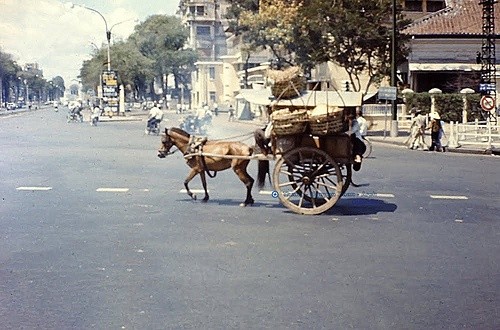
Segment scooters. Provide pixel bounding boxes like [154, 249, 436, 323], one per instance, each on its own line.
[185, 117, 207, 136]
[90, 113, 98, 126]
[145, 116, 160, 136]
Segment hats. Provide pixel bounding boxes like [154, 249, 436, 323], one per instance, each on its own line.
[429, 112, 441, 120]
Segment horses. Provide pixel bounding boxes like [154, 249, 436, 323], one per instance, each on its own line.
[158, 127, 267, 206]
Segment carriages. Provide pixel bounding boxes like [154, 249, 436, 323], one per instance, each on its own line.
[157, 127, 354, 214]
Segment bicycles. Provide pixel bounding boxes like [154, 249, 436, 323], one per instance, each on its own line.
[361, 137, 372, 158]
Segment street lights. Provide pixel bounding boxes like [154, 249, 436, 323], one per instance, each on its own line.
[67, 2, 139, 71]
[18, 74, 39, 101]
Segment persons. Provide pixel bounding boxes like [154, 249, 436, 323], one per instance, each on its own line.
[406, 108, 447, 156]
[227, 105, 235, 122]
[214, 102, 218, 116]
[357, 112, 367, 136]
[345, 116, 363, 163]
[2, 90, 214, 136]
